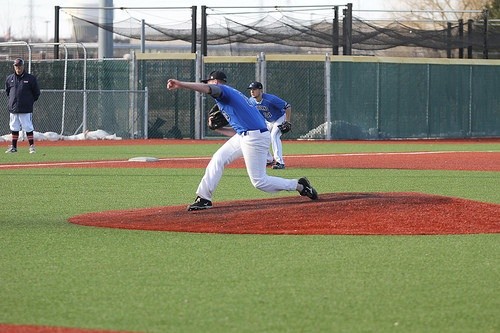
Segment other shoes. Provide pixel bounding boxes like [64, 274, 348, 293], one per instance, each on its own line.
[266, 160, 275, 166]
[29, 144, 36, 153]
[272, 162, 284, 168]
[5, 144, 17, 154]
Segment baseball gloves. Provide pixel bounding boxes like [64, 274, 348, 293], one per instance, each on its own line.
[207, 111, 229, 131]
[276, 120, 292, 134]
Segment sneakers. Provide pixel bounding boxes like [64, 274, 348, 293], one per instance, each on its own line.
[298, 177, 318, 200]
[186, 195, 212, 210]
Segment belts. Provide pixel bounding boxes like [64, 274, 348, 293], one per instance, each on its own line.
[241, 127, 268, 135]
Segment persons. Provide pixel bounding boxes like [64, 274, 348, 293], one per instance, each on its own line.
[247, 81, 291, 169]
[5, 58, 40, 154]
[167, 70, 318, 211]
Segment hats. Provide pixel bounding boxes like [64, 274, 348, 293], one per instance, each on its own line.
[12, 58, 24, 66]
[247, 82, 262, 89]
[201, 70, 227, 85]
